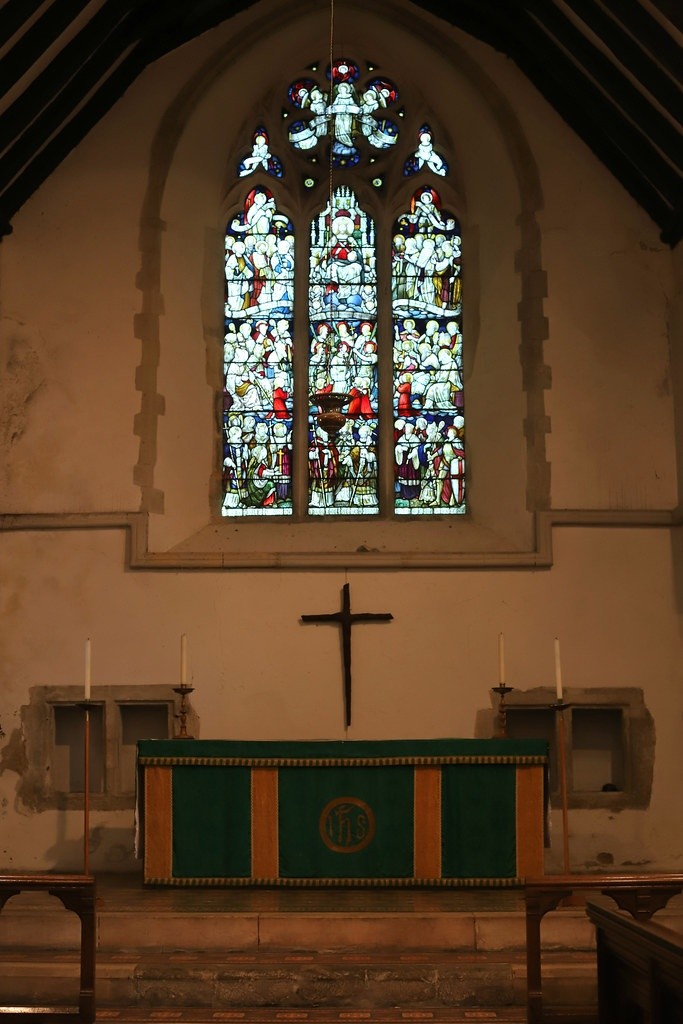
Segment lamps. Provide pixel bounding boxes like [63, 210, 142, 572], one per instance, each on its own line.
[311, 0, 353, 439]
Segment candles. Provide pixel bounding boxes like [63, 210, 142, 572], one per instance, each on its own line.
[181, 633, 187, 684]
[498, 633, 504, 684]
[85, 637, 90, 699]
[554, 638, 562, 698]
